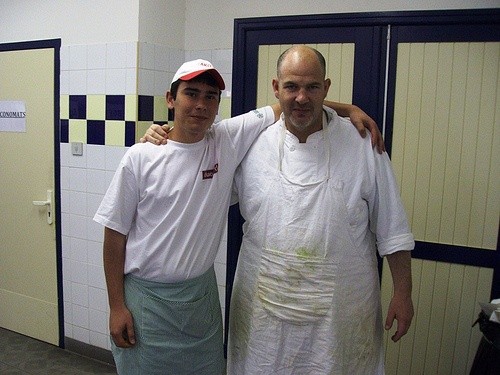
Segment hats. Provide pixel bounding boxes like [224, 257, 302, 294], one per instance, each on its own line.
[169, 59, 226, 94]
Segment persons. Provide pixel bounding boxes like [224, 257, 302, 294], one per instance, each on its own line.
[93, 59, 415, 375]
[140, 45, 415, 375]
